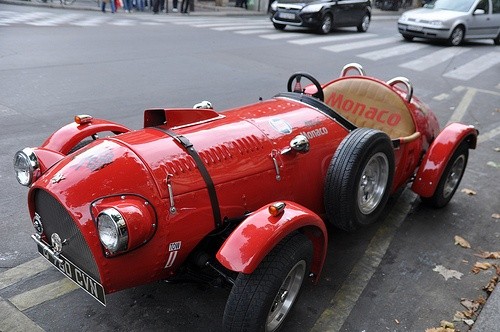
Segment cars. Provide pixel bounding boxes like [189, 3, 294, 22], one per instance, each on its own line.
[398, 1, 500, 47]
[271, 0, 372, 35]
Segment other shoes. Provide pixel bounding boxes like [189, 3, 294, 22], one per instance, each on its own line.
[173, 8, 178, 12]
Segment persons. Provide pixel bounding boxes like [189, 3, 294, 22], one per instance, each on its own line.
[101, 0, 195, 17]
